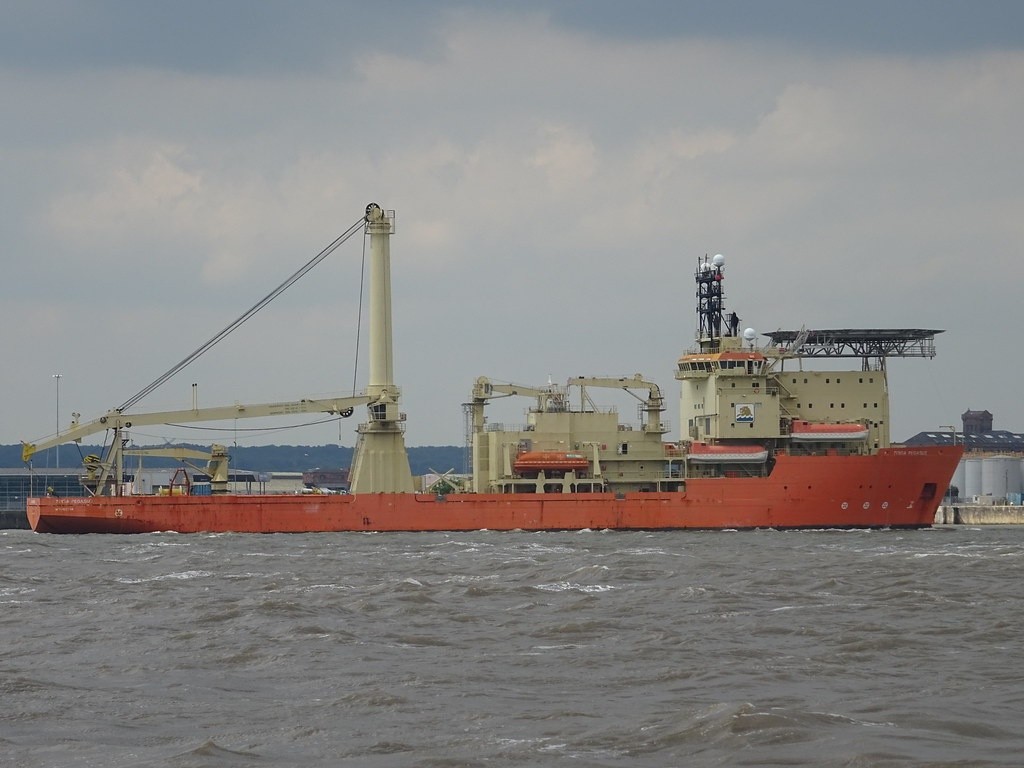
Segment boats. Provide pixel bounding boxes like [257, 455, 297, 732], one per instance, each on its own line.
[25, 200, 965, 533]
